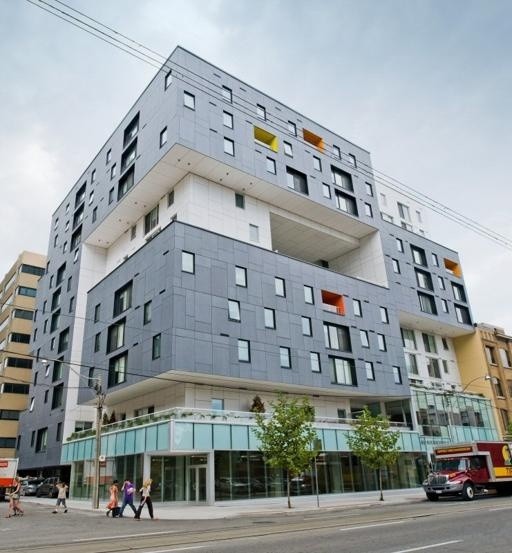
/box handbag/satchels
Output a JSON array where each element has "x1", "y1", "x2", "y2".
[{"x1": 12, "y1": 493, "x2": 19, "y2": 498}]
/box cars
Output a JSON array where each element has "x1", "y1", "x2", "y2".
[
  {"x1": 219, "y1": 476, "x2": 324, "y2": 495},
  {"x1": 21, "y1": 476, "x2": 45, "y2": 496},
  {"x1": 36, "y1": 476, "x2": 66, "y2": 498}
]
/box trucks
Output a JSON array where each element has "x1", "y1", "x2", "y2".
[{"x1": 421, "y1": 440, "x2": 512, "y2": 502}]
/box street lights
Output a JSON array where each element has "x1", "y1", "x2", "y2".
[{"x1": 40, "y1": 359, "x2": 106, "y2": 510}]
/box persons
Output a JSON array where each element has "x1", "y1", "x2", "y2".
[
  {"x1": 134, "y1": 479, "x2": 156, "y2": 519},
  {"x1": 115, "y1": 478, "x2": 137, "y2": 517},
  {"x1": 51, "y1": 481, "x2": 68, "y2": 512},
  {"x1": 8, "y1": 475, "x2": 24, "y2": 516},
  {"x1": 104, "y1": 479, "x2": 119, "y2": 517}
]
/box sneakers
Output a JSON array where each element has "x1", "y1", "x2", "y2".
[
  {"x1": 64, "y1": 509, "x2": 67, "y2": 512},
  {"x1": 52, "y1": 510, "x2": 57, "y2": 512}
]
